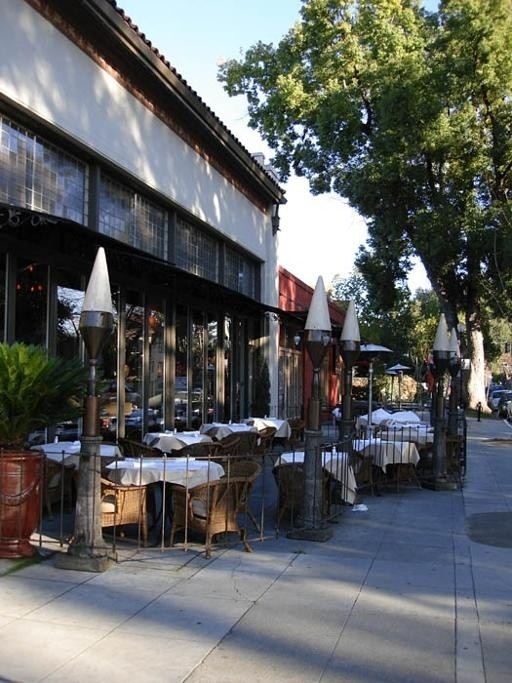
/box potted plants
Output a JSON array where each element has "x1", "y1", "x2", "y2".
[{"x1": 0, "y1": 343, "x2": 105, "y2": 558}]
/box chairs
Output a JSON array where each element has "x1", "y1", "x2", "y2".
[
  {"x1": 219, "y1": 463, "x2": 263, "y2": 538},
  {"x1": 169, "y1": 477, "x2": 255, "y2": 561},
  {"x1": 271, "y1": 462, "x2": 334, "y2": 529},
  {"x1": 73, "y1": 468, "x2": 148, "y2": 553},
  {"x1": 32, "y1": 411, "x2": 433, "y2": 498}
]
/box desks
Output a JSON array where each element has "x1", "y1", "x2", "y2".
[
  {"x1": 273, "y1": 451, "x2": 359, "y2": 507},
  {"x1": 104, "y1": 459, "x2": 227, "y2": 547}
]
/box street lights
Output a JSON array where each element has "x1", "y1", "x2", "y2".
[
  {"x1": 340, "y1": 298, "x2": 360, "y2": 437},
  {"x1": 288, "y1": 275, "x2": 332, "y2": 541},
  {"x1": 52, "y1": 243, "x2": 116, "y2": 574},
  {"x1": 433, "y1": 312, "x2": 460, "y2": 491}
]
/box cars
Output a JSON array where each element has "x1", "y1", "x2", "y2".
[
  {"x1": 487, "y1": 386, "x2": 512, "y2": 421},
  {"x1": 109, "y1": 407, "x2": 158, "y2": 430},
  {"x1": 174, "y1": 390, "x2": 203, "y2": 428},
  {"x1": 28, "y1": 424, "x2": 78, "y2": 446}
]
